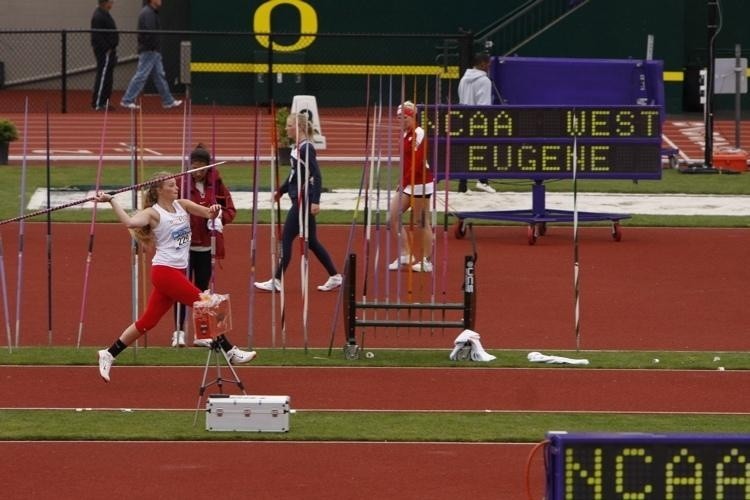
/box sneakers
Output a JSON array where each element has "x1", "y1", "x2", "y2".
[
  {"x1": 96, "y1": 347, "x2": 116, "y2": 384},
  {"x1": 475, "y1": 180, "x2": 498, "y2": 195},
  {"x1": 169, "y1": 331, "x2": 187, "y2": 348},
  {"x1": 317, "y1": 272, "x2": 343, "y2": 293},
  {"x1": 456, "y1": 189, "x2": 478, "y2": 197},
  {"x1": 161, "y1": 99, "x2": 183, "y2": 109},
  {"x1": 226, "y1": 345, "x2": 257, "y2": 365},
  {"x1": 411, "y1": 257, "x2": 433, "y2": 273},
  {"x1": 193, "y1": 337, "x2": 214, "y2": 348},
  {"x1": 89, "y1": 102, "x2": 116, "y2": 112},
  {"x1": 388, "y1": 253, "x2": 415, "y2": 271},
  {"x1": 253, "y1": 278, "x2": 282, "y2": 293},
  {"x1": 120, "y1": 100, "x2": 141, "y2": 109}
]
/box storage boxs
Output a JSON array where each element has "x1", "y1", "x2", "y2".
[{"x1": 205, "y1": 394, "x2": 291, "y2": 433}]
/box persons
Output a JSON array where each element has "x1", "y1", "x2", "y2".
[
  {"x1": 483, "y1": 34, "x2": 498, "y2": 57},
  {"x1": 458, "y1": 52, "x2": 496, "y2": 194},
  {"x1": 254, "y1": 113, "x2": 344, "y2": 294},
  {"x1": 389, "y1": 101, "x2": 434, "y2": 273},
  {"x1": 172, "y1": 142, "x2": 236, "y2": 348},
  {"x1": 119, "y1": 0, "x2": 183, "y2": 113},
  {"x1": 89, "y1": 1, "x2": 119, "y2": 113},
  {"x1": 90, "y1": 171, "x2": 257, "y2": 384}
]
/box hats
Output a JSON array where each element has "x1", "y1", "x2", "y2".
[{"x1": 397, "y1": 100, "x2": 418, "y2": 118}]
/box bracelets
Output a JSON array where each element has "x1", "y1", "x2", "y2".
[{"x1": 108, "y1": 197, "x2": 115, "y2": 203}]
